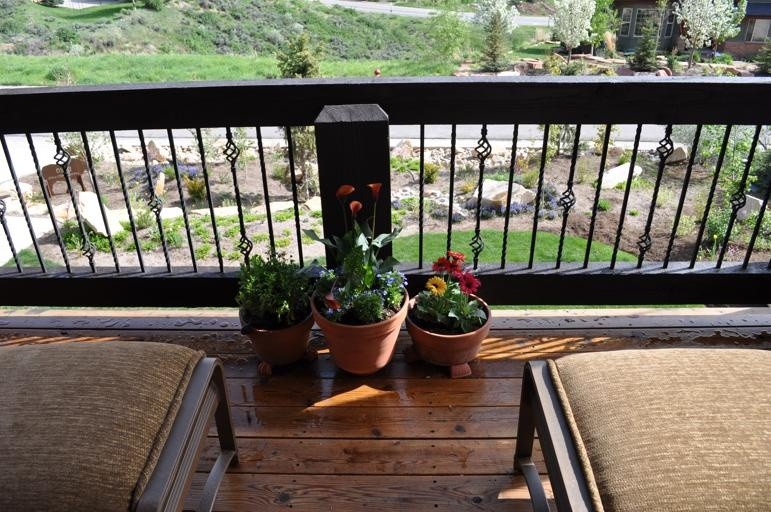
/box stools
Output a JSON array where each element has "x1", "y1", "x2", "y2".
[
  {"x1": 514, "y1": 348, "x2": 771, "y2": 511},
  {"x1": 0, "y1": 342, "x2": 238, "y2": 512}
]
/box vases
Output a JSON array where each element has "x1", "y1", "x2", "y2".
[
  {"x1": 310, "y1": 282, "x2": 407, "y2": 373},
  {"x1": 405, "y1": 290, "x2": 491, "y2": 366}
]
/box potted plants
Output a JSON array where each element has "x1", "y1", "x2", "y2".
[{"x1": 236, "y1": 250, "x2": 312, "y2": 369}]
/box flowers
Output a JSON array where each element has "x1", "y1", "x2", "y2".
[
  {"x1": 413, "y1": 251, "x2": 487, "y2": 330},
  {"x1": 316, "y1": 182, "x2": 408, "y2": 324}
]
